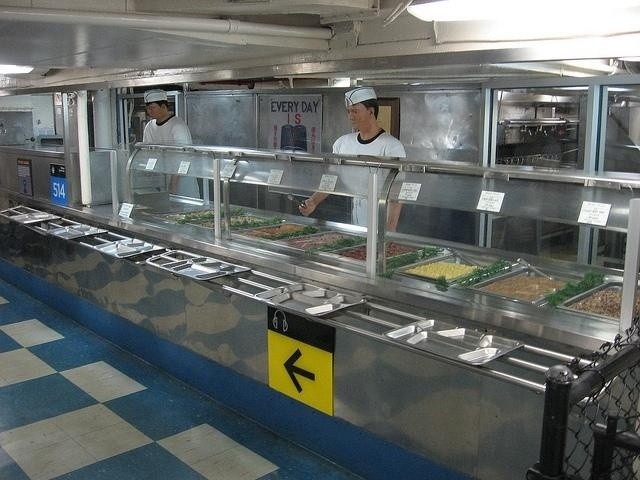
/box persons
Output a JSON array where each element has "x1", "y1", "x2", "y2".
[
  {"x1": 142, "y1": 89, "x2": 200, "y2": 199},
  {"x1": 298, "y1": 86, "x2": 407, "y2": 231}
]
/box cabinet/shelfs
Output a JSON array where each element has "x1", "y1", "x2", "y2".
[{"x1": 123, "y1": 141, "x2": 640, "y2": 349}]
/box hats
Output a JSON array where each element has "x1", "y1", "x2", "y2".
[
  {"x1": 345, "y1": 86, "x2": 378, "y2": 109},
  {"x1": 143, "y1": 89, "x2": 168, "y2": 104}
]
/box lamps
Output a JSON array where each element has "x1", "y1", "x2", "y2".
[{"x1": 0, "y1": 63, "x2": 34, "y2": 74}]
